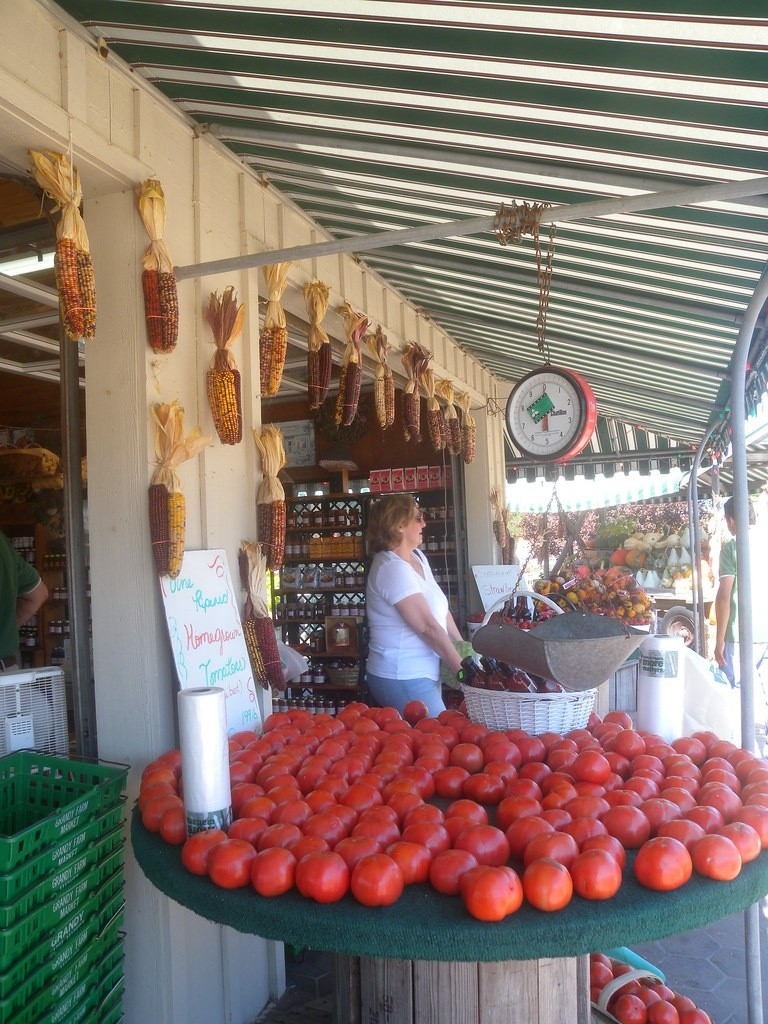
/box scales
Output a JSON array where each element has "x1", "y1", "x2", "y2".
[{"x1": 470, "y1": 362, "x2": 652, "y2": 691}]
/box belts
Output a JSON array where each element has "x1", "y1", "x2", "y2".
[{"x1": 0, "y1": 655, "x2": 17, "y2": 672}]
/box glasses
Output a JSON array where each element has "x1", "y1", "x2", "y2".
[{"x1": 411, "y1": 512, "x2": 423, "y2": 524}]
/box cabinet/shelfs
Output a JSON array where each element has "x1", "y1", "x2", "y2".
[
  {"x1": 271, "y1": 485, "x2": 467, "y2": 692},
  {"x1": 0, "y1": 522, "x2": 92, "y2": 669}
]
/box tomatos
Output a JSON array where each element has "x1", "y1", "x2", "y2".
[
  {"x1": 588, "y1": 952, "x2": 712, "y2": 1024},
  {"x1": 468, "y1": 611, "x2": 651, "y2": 629},
  {"x1": 138, "y1": 699, "x2": 768, "y2": 921}
]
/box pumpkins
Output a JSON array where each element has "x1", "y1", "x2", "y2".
[{"x1": 532, "y1": 525, "x2": 711, "y2": 617}]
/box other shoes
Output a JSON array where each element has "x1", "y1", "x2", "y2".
[{"x1": 754, "y1": 723, "x2": 768, "y2": 758}]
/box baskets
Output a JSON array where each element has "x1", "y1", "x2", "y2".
[
  {"x1": 456, "y1": 678, "x2": 597, "y2": 737},
  {"x1": 0, "y1": 748, "x2": 132, "y2": 1024}
]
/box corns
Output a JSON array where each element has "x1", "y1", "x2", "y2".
[
  {"x1": 148, "y1": 483, "x2": 186, "y2": 579},
  {"x1": 256, "y1": 500, "x2": 286, "y2": 571},
  {"x1": 242, "y1": 616, "x2": 287, "y2": 692},
  {"x1": 53, "y1": 237, "x2": 477, "y2": 465},
  {"x1": 493, "y1": 520, "x2": 515, "y2": 565}
]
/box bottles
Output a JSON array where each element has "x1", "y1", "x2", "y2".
[
  {"x1": 457, "y1": 654, "x2": 567, "y2": 701},
  {"x1": 503, "y1": 596, "x2": 531, "y2": 622}
]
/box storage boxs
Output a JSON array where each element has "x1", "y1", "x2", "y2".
[
  {"x1": 442, "y1": 465, "x2": 453, "y2": 488},
  {"x1": 429, "y1": 466, "x2": 442, "y2": 489},
  {"x1": 381, "y1": 468, "x2": 393, "y2": 493},
  {"x1": 405, "y1": 467, "x2": 417, "y2": 490},
  {"x1": 417, "y1": 465, "x2": 430, "y2": 489},
  {"x1": 0, "y1": 747, "x2": 133, "y2": 1024},
  {"x1": 392, "y1": 468, "x2": 405, "y2": 492},
  {"x1": 369, "y1": 470, "x2": 381, "y2": 492}
]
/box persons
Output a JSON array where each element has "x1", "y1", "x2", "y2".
[
  {"x1": 0, "y1": 530, "x2": 48, "y2": 671},
  {"x1": 715, "y1": 496, "x2": 768, "y2": 689},
  {"x1": 366, "y1": 493, "x2": 466, "y2": 719}
]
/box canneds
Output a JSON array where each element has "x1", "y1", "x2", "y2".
[
  {"x1": 6, "y1": 536, "x2": 69, "y2": 658},
  {"x1": 273, "y1": 504, "x2": 457, "y2": 717}
]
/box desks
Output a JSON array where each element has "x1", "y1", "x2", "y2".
[{"x1": 131, "y1": 798, "x2": 768, "y2": 1024}]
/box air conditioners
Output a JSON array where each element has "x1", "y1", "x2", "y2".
[{"x1": 0, "y1": 666, "x2": 70, "y2": 778}]
